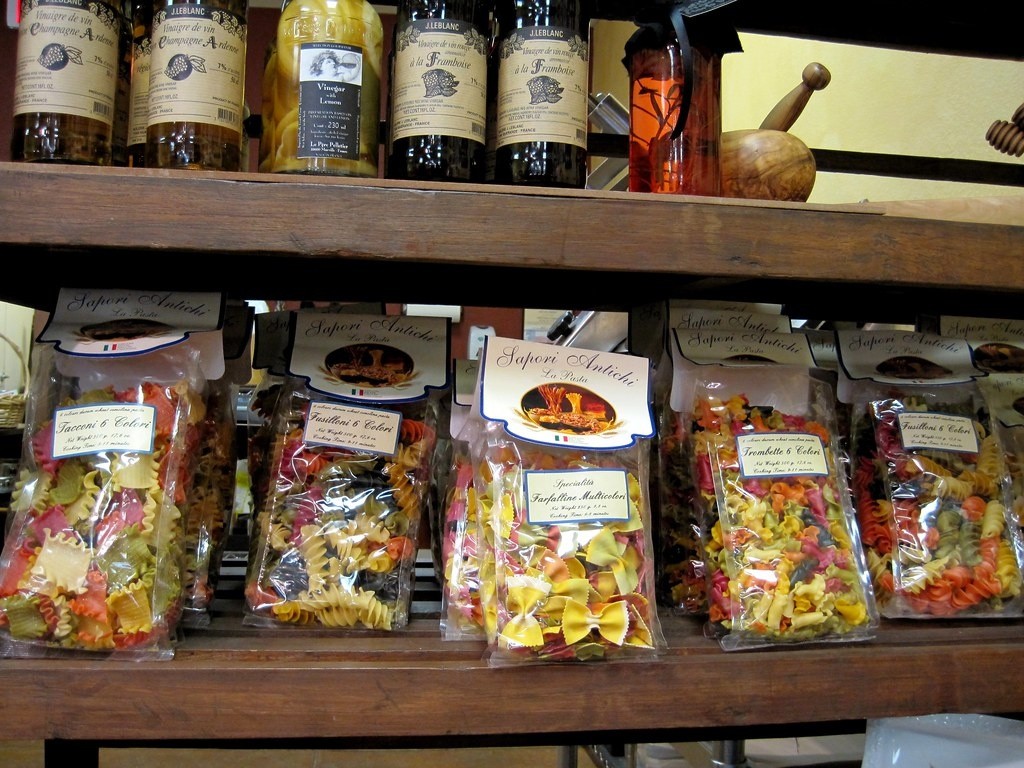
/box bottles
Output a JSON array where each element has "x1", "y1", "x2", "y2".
[
  {"x1": 388, "y1": 0, "x2": 488, "y2": 182},
  {"x1": 256, "y1": 0, "x2": 384, "y2": 175},
  {"x1": 11, "y1": 0, "x2": 246, "y2": 178},
  {"x1": 498, "y1": 0, "x2": 590, "y2": 188},
  {"x1": 629, "y1": 28, "x2": 722, "y2": 197}
]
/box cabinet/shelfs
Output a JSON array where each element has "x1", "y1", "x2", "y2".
[{"x1": 0, "y1": 160, "x2": 1024, "y2": 738}]
